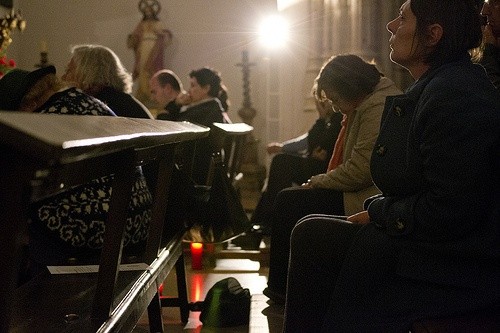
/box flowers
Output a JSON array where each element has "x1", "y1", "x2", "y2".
[{"x1": 0, "y1": 55, "x2": 16, "y2": 78}]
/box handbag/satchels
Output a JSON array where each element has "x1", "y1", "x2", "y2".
[{"x1": 198, "y1": 277, "x2": 252, "y2": 328}]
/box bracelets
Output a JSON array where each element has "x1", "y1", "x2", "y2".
[
  {"x1": 308, "y1": 178, "x2": 314, "y2": 190},
  {"x1": 302, "y1": 182, "x2": 306, "y2": 188}
]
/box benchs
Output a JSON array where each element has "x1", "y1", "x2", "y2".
[{"x1": 0, "y1": 110, "x2": 255, "y2": 333}]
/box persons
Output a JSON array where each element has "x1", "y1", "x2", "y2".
[
  {"x1": 154, "y1": 67, "x2": 231, "y2": 187},
  {"x1": 149, "y1": 69, "x2": 185, "y2": 108},
  {"x1": 0, "y1": 65, "x2": 153, "y2": 309},
  {"x1": 262, "y1": 53, "x2": 402, "y2": 307},
  {"x1": 283, "y1": 0, "x2": 500, "y2": 333},
  {"x1": 127, "y1": 4, "x2": 173, "y2": 108},
  {"x1": 480, "y1": 0, "x2": 500, "y2": 90},
  {"x1": 232, "y1": 84, "x2": 344, "y2": 253},
  {"x1": 58, "y1": 43, "x2": 190, "y2": 238}
]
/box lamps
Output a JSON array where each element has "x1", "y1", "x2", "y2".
[{"x1": 190, "y1": 242, "x2": 204, "y2": 271}]
